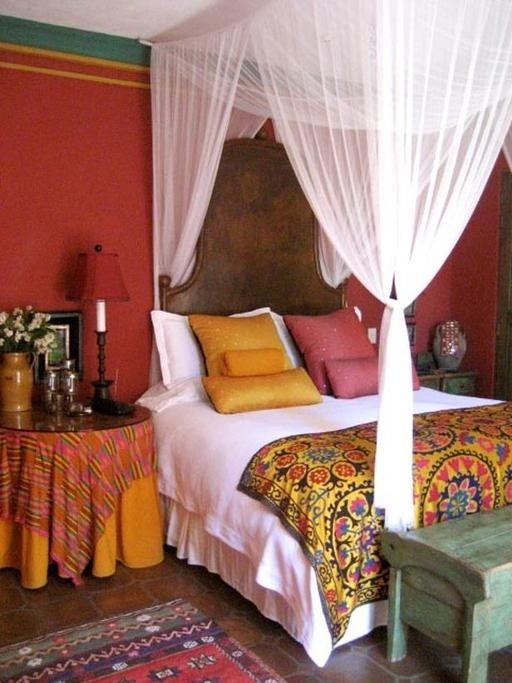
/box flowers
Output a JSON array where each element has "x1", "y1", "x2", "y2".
[{"x1": 0, "y1": 303, "x2": 62, "y2": 356}]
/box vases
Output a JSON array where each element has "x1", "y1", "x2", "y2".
[
  {"x1": 1, "y1": 350, "x2": 37, "y2": 413},
  {"x1": 431, "y1": 319, "x2": 468, "y2": 373}
]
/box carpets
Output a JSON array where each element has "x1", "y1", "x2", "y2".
[{"x1": 0, "y1": 597, "x2": 287, "y2": 683}]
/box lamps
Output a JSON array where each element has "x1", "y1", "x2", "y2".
[{"x1": 66, "y1": 242, "x2": 137, "y2": 415}]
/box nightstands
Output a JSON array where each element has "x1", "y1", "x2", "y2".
[{"x1": 417, "y1": 370, "x2": 480, "y2": 396}]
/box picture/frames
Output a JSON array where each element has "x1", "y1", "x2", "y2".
[
  {"x1": 406, "y1": 324, "x2": 416, "y2": 346},
  {"x1": 32, "y1": 310, "x2": 84, "y2": 386},
  {"x1": 405, "y1": 300, "x2": 416, "y2": 317}
]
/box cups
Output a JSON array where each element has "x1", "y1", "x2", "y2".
[{"x1": 51, "y1": 393, "x2": 71, "y2": 415}]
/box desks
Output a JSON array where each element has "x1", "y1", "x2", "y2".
[{"x1": 0, "y1": 398, "x2": 167, "y2": 589}]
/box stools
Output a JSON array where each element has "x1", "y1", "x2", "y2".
[{"x1": 378, "y1": 504, "x2": 512, "y2": 683}]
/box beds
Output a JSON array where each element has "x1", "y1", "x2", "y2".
[{"x1": 133, "y1": 137, "x2": 511, "y2": 670}]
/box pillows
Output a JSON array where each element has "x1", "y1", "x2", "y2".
[{"x1": 149, "y1": 306, "x2": 421, "y2": 415}]
